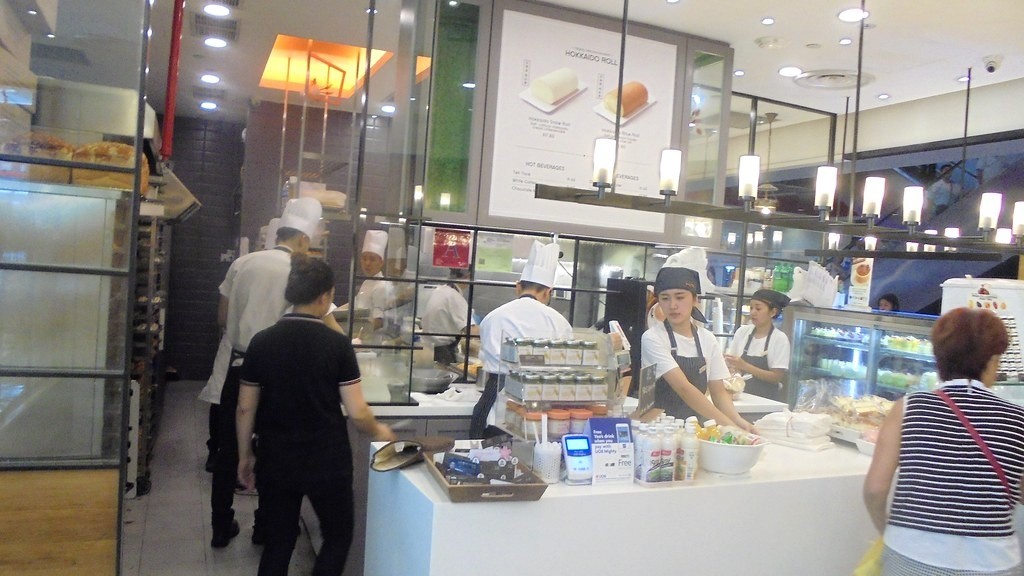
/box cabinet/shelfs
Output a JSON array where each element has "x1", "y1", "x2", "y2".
[
  {"x1": 301, "y1": 419, "x2": 472, "y2": 576},
  {"x1": 496, "y1": 328, "x2": 614, "y2": 439},
  {"x1": 102, "y1": 215, "x2": 165, "y2": 494}
]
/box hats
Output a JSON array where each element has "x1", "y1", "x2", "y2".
[
  {"x1": 519, "y1": 240, "x2": 560, "y2": 289},
  {"x1": 362, "y1": 229, "x2": 388, "y2": 261},
  {"x1": 277, "y1": 197, "x2": 321, "y2": 241},
  {"x1": 387, "y1": 228, "x2": 407, "y2": 260}
]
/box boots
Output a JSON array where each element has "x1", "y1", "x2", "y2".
[
  {"x1": 205, "y1": 439, "x2": 216, "y2": 472},
  {"x1": 253, "y1": 508, "x2": 301, "y2": 544},
  {"x1": 211, "y1": 508, "x2": 239, "y2": 547}
]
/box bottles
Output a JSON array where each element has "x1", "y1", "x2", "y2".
[
  {"x1": 630, "y1": 415, "x2": 763, "y2": 482},
  {"x1": 745, "y1": 262, "x2": 794, "y2": 293}
]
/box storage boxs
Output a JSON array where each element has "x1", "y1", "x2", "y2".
[
  {"x1": 299, "y1": 181, "x2": 346, "y2": 208},
  {"x1": 0, "y1": 0, "x2": 58, "y2": 178}
]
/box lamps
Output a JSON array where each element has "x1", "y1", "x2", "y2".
[
  {"x1": 705, "y1": 156, "x2": 760, "y2": 212},
  {"x1": 878, "y1": 186, "x2": 923, "y2": 235},
  {"x1": 927, "y1": 193, "x2": 1002, "y2": 242},
  {"x1": 964, "y1": 201, "x2": 1024, "y2": 245},
  {"x1": 755, "y1": 113, "x2": 778, "y2": 215},
  {"x1": 769, "y1": 167, "x2": 837, "y2": 221},
  {"x1": 829, "y1": 177, "x2": 885, "y2": 228},
  {"x1": 726, "y1": 227, "x2": 961, "y2": 256},
  {"x1": 650, "y1": 150, "x2": 682, "y2": 206},
  {"x1": 577, "y1": 138, "x2": 616, "y2": 199}
]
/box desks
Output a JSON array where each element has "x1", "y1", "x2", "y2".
[{"x1": 709, "y1": 389, "x2": 790, "y2": 421}]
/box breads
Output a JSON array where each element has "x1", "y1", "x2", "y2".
[
  {"x1": 832, "y1": 394, "x2": 896, "y2": 430},
  {"x1": 534, "y1": 68, "x2": 579, "y2": 105},
  {"x1": 0, "y1": 130, "x2": 149, "y2": 194},
  {"x1": 606, "y1": 82, "x2": 648, "y2": 116}
]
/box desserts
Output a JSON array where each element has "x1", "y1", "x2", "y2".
[{"x1": 809, "y1": 326, "x2": 933, "y2": 387}]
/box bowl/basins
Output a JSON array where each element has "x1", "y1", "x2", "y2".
[{"x1": 698, "y1": 439, "x2": 764, "y2": 474}]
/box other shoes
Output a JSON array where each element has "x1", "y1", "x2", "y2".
[{"x1": 235, "y1": 488, "x2": 259, "y2": 496}]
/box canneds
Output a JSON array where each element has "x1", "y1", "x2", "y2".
[
  {"x1": 504, "y1": 337, "x2": 599, "y2": 366},
  {"x1": 504, "y1": 370, "x2": 607, "y2": 402},
  {"x1": 504, "y1": 400, "x2": 607, "y2": 438}
]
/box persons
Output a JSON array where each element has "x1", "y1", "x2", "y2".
[
  {"x1": 198, "y1": 197, "x2": 349, "y2": 549},
  {"x1": 204, "y1": 217, "x2": 282, "y2": 495},
  {"x1": 863, "y1": 306, "x2": 1023, "y2": 576},
  {"x1": 647, "y1": 247, "x2": 716, "y2": 329},
  {"x1": 470, "y1": 240, "x2": 572, "y2": 440},
  {"x1": 918, "y1": 163, "x2": 937, "y2": 185},
  {"x1": 724, "y1": 289, "x2": 791, "y2": 424},
  {"x1": 641, "y1": 267, "x2": 762, "y2": 437},
  {"x1": 256, "y1": 218, "x2": 281, "y2": 250},
  {"x1": 926, "y1": 164, "x2": 962, "y2": 222},
  {"x1": 238, "y1": 252, "x2": 399, "y2": 576},
  {"x1": 382, "y1": 225, "x2": 417, "y2": 346},
  {"x1": 331, "y1": 230, "x2": 399, "y2": 337},
  {"x1": 878, "y1": 293, "x2": 903, "y2": 401},
  {"x1": 421, "y1": 264, "x2": 480, "y2": 364}
]
